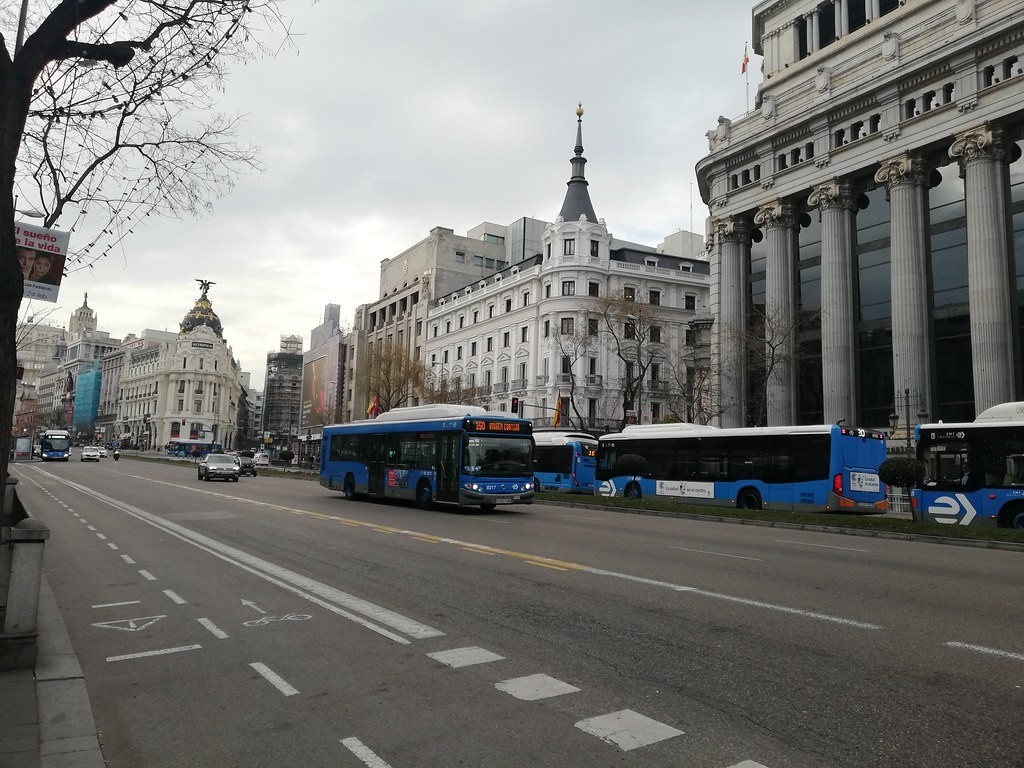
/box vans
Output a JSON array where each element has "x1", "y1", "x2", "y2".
[{"x1": 254, "y1": 453, "x2": 269, "y2": 467}]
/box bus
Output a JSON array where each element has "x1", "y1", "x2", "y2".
[
  {"x1": 40, "y1": 430, "x2": 69, "y2": 462},
  {"x1": 167, "y1": 439, "x2": 222, "y2": 457},
  {"x1": 532, "y1": 432, "x2": 597, "y2": 496},
  {"x1": 593, "y1": 422, "x2": 888, "y2": 515},
  {"x1": 319, "y1": 403, "x2": 535, "y2": 512},
  {"x1": 913, "y1": 401, "x2": 1024, "y2": 532}
]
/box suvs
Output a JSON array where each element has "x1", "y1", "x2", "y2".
[
  {"x1": 80, "y1": 447, "x2": 101, "y2": 462},
  {"x1": 98, "y1": 447, "x2": 107, "y2": 457}
]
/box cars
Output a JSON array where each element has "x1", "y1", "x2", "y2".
[{"x1": 198, "y1": 454, "x2": 239, "y2": 482}]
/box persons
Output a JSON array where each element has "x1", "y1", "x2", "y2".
[
  {"x1": 26, "y1": 428, "x2": 325, "y2": 465},
  {"x1": 16, "y1": 245, "x2": 35, "y2": 281},
  {"x1": 29, "y1": 250, "x2": 58, "y2": 286}
]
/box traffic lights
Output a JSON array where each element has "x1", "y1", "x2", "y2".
[{"x1": 182, "y1": 419, "x2": 185, "y2": 425}]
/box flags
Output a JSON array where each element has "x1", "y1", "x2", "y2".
[{"x1": 741, "y1": 45, "x2": 750, "y2": 75}]
